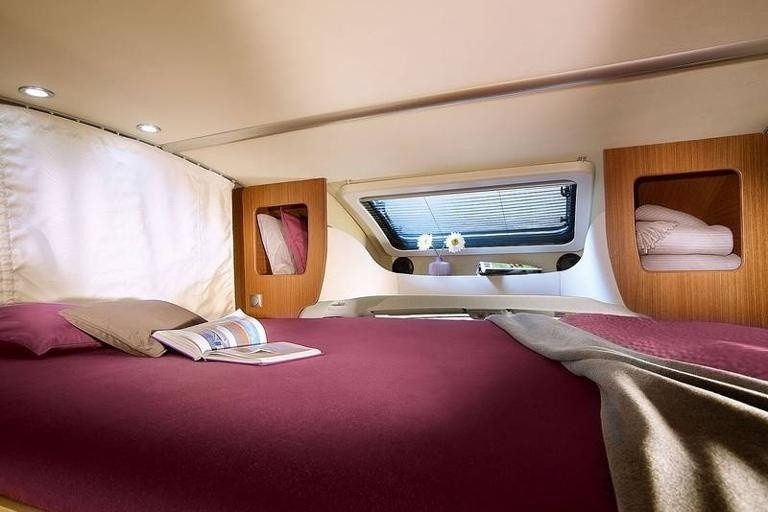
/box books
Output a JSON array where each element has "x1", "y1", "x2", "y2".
[
  {"x1": 476, "y1": 261, "x2": 544, "y2": 275},
  {"x1": 150, "y1": 307, "x2": 323, "y2": 366}
]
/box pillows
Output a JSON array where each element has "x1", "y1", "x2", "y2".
[
  {"x1": 0, "y1": 299, "x2": 209, "y2": 357},
  {"x1": 256, "y1": 209, "x2": 308, "y2": 274}
]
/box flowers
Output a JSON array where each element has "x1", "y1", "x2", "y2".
[{"x1": 416, "y1": 232, "x2": 465, "y2": 257}]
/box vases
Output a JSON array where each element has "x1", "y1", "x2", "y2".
[{"x1": 428, "y1": 257, "x2": 449, "y2": 275}]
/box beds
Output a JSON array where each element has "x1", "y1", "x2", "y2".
[{"x1": 0, "y1": 315, "x2": 767, "y2": 512}]
